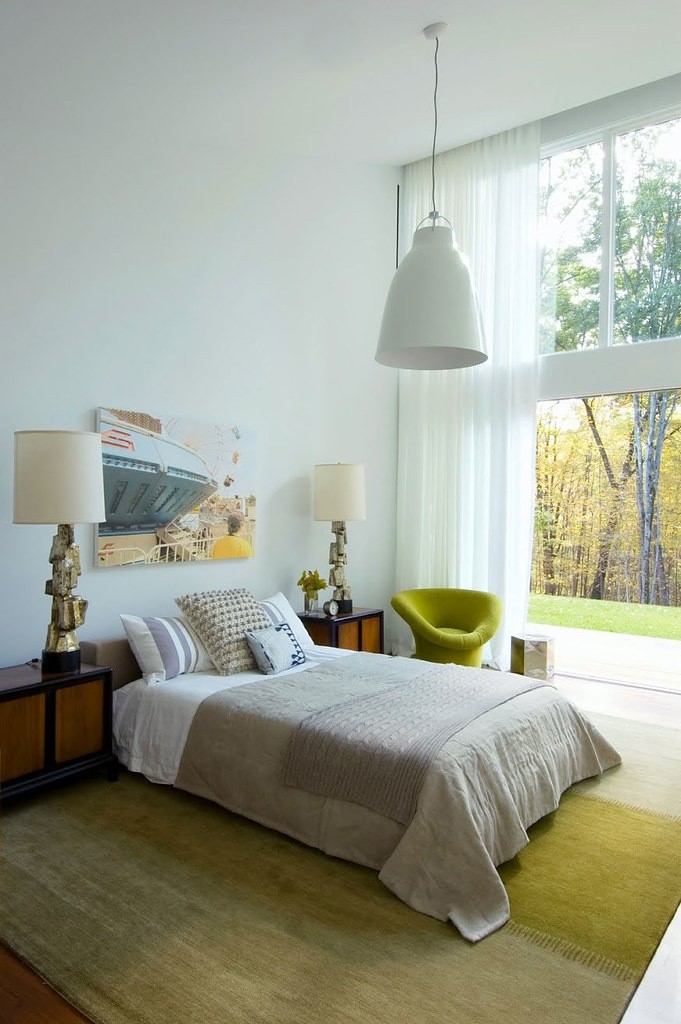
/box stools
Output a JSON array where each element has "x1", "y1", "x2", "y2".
[{"x1": 511, "y1": 634, "x2": 553, "y2": 682}]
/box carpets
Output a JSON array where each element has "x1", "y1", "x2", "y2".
[{"x1": 0, "y1": 706, "x2": 679, "y2": 1024}]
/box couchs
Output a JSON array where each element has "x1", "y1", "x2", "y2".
[{"x1": 391, "y1": 590, "x2": 502, "y2": 669}]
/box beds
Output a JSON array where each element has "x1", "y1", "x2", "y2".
[{"x1": 79, "y1": 633, "x2": 622, "y2": 943}]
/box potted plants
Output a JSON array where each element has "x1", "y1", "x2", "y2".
[{"x1": 296, "y1": 570, "x2": 327, "y2": 614}]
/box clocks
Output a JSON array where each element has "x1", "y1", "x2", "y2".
[{"x1": 323, "y1": 601, "x2": 338, "y2": 615}]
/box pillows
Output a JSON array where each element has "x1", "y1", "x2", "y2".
[
  {"x1": 117, "y1": 614, "x2": 218, "y2": 684},
  {"x1": 245, "y1": 620, "x2": 306, "y2": 676},
  {"x1": 174, "y1": 589, "x2": 272, "y2": 675},
  {"x1": 258, "y1": 592, "x2": 313, "y2": 649}
]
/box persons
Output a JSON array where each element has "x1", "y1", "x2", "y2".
[{"x1": 212, "y1": 510, "x2": 252, "y2": 560}]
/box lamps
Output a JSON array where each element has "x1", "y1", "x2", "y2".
[
  {"x1": 12, "y1": 429, "x2": 107, "y2": 673},
  {"x1": 373, "y1": 21, "x2": 489, "y2": 371},
  {"x1": 312, "y1": 463, "x2": 367, "y2": 613}
]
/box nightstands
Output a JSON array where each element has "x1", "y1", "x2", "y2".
[
  {"x1": 0, "y1": 660, "x2": 121, "y2": 808},
  {"x1": 295, "y1": 604, "x2": 386, "y2": 654}
]
